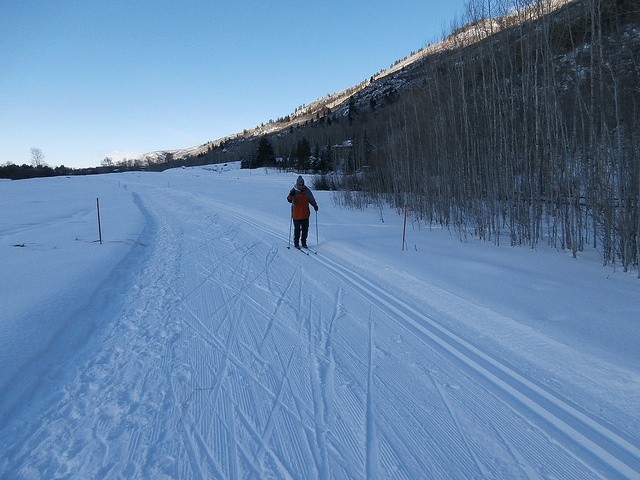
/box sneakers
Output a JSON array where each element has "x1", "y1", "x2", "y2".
[
  {"x1": 294, "y1": 245, "x2": 301, "y2": 250},
  {"x1": 302, "y1": 244, "x2": 308, "y2": 248}
]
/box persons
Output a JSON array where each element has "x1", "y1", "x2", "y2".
[{"x1": 287, "y1": 175, "x2": 318, "y2": 249}]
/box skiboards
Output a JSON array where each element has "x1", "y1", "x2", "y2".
[{"x1": 294, "y1": 245, "x2": 317, "y2": 255}]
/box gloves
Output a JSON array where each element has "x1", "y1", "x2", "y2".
[
  {"x1": 314, "y1": 206, "x2": 318, "y2": 211},
  {"x1": 290, "y1": 190, "x2": 295, "y2": 196}
]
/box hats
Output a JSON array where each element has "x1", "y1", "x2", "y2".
[{"x1": 297, "y1": 176, "x2": 304, "y2": 183}]
